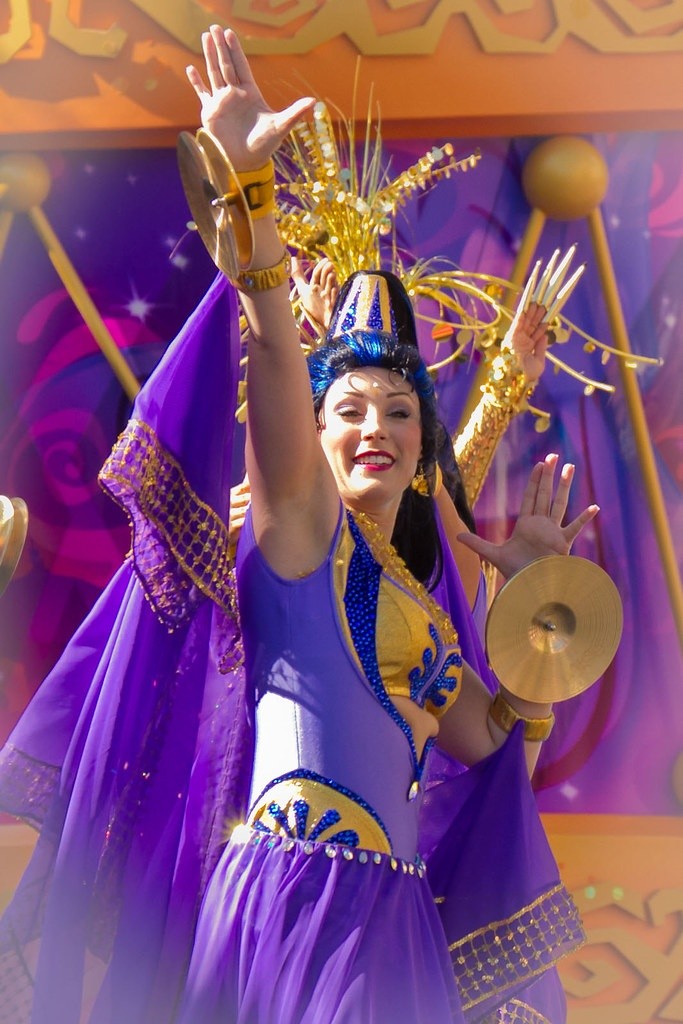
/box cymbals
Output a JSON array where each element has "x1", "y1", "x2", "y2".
[
  {"x1": 485, "y1": 555, "x2": 625, "y2": 704},
  {"x1": 0, "y1": 495, "x2": 30, "y2": 594},
  {"x1": 175, "y1": 129, "x2": 258, "y2": 277}
]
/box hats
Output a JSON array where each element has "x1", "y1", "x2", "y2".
[{"x1": 325, "y1": 266, "x2": 418, "y2": 359}]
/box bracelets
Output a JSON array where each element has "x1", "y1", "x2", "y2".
[
  {"x1": 488, "y1": 693, "x2": 556, "y2": 742},
  {"x1": 226, "y1": 252, "x2": 296, "y2": 293}
]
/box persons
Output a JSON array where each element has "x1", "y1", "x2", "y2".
[
  {"x1": 182, "y1": 22, "x2": 606, "y2": 1024},
  {"x1": 290, "y1": 253, "x2": 557, "y2": 510}
]
